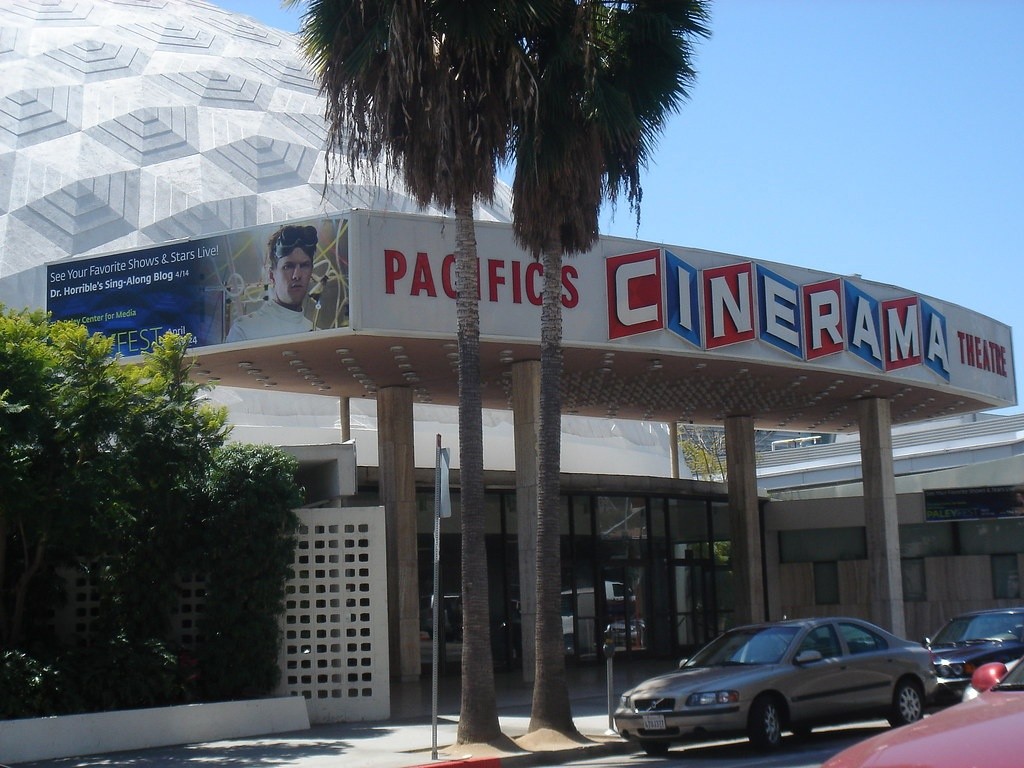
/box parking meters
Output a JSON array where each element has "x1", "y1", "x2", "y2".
[{"x1": 603, "y1": 624, "x2": 616, "y2": 728}]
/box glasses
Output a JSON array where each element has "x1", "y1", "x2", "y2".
[{"x1": 275, "y1": 226, "x2": 318, "y2": 257}]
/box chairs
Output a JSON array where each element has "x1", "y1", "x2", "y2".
[{"x1": 816, "y1": 636, "x2": 839, "y2": 657}]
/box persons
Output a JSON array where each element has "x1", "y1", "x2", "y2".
[{"x1": 223, "y1": 225, "x2": 324, "y2": 343}]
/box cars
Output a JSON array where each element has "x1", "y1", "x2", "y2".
[
  {"x1": 921, "y1": 607, "x2": 1024, "y2": 693},
  {"x1": 823, "y1": 659, "x2": 1024, "y2": 768},
  {"x1": 613, "y1": 616, "x2": 938, "y2": 755}
]
